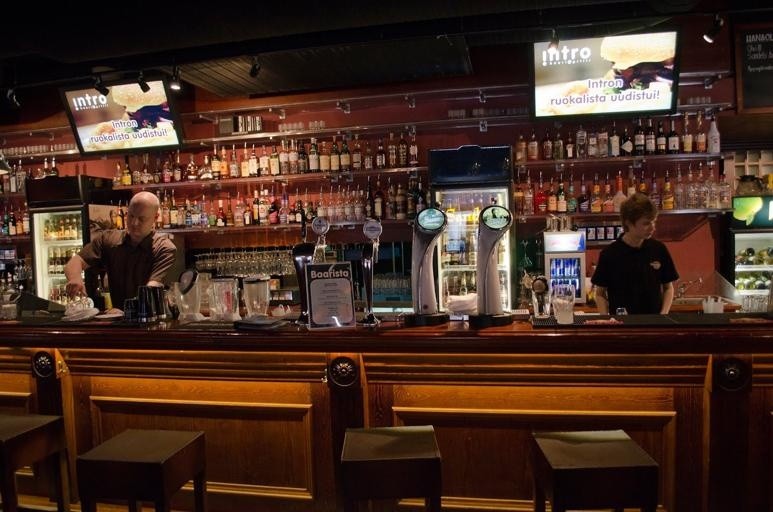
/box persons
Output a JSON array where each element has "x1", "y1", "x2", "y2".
[
  {"x1": 62, "y1": 191, "x2": 178, "y2": 315},
  {"x1": 591, "y1": 193, "x2": 680, "y2": 316}
]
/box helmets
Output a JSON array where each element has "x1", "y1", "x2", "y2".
[{"x1": 93, "y1": 314, "x2": 122, "y2": 322}]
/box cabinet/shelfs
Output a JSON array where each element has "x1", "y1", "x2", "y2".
[
  {"x1": 123, "y1": 285, "x2": 166, "y2": 326},
  {"x1": 42, "y1": 157, "x2": 49, "y2": 178},
  {"x1": 515, "y1": 110, "x2": 731, "y2": 213},
  {"x1": 0, "y1": 159, "x2": 41, "y2": 293},
  {"x1": 49, "y1": 156, "x2": 58, "y2": 176},
  {"x1": 44, "y1": 214, "x2": 84, "y2": 305},
  {"x1": 436, "y1": 195, "x2": 507, "y2": 304}
]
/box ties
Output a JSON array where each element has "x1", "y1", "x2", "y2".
[
  {"x1": 59, "y1": 72, "x2": 183, "y2": 155},
  {"x1": 528, "y1": 27, "x2": 681, "y2": 119}
]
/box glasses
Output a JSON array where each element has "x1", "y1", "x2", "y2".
[{"x1": 0, "y1": 7, "x2": 726, "y2": 110}]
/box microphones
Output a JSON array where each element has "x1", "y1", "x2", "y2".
[
  {"x1": 335, "y1": 423, "x2": 444, "y2": 511},
  {"x1": 0, "y1": 408, "x2": 73, "y2": 511},
  {"x1": 71, "y1": 426, "x2": 211, "y2": 511},
  {"x1": 526, "y1": 425, "x2": 664, "y2": 512}
]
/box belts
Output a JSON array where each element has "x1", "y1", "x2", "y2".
[{"x1": 192, "y1": 248, "x2": 298, "y2": 280}]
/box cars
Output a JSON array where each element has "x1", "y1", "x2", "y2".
[
  {"x1": 172, "y1": 277, "x2": 271, "y2": 323},
  {"x1": 742, "y1": 293, "x2": 768, "y2": 312},
  {"x1": 545, "y1": 217, "x2": 574, "y2": 232},
  {"x1": 531, "y1": 275, "x2": 576, "y2": 325}
]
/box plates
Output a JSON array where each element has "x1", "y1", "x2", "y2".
[
  {"x1": 0, "y1": 191, "x2": 30, "y2": 247},
  {"x1": 105, "y1": 164, "x2": 430, "y2": 236},
  {"x1": 508, "y1": 150, "x2": 739, "y2": 221}
]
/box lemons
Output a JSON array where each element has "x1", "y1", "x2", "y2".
[{"x1": 736, "y1": 173, "x2": 765, "y2": 196}]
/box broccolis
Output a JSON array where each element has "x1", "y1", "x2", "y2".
[
  {"x1": 720, "y1": 194, "x2": 772, "y2": 314},
  {"x1": 23, "y1": 174, "x2": 112, "y2": 299},
  {"x1": 423, "y1": 147, "x2": 514, "y2": 312}
]
